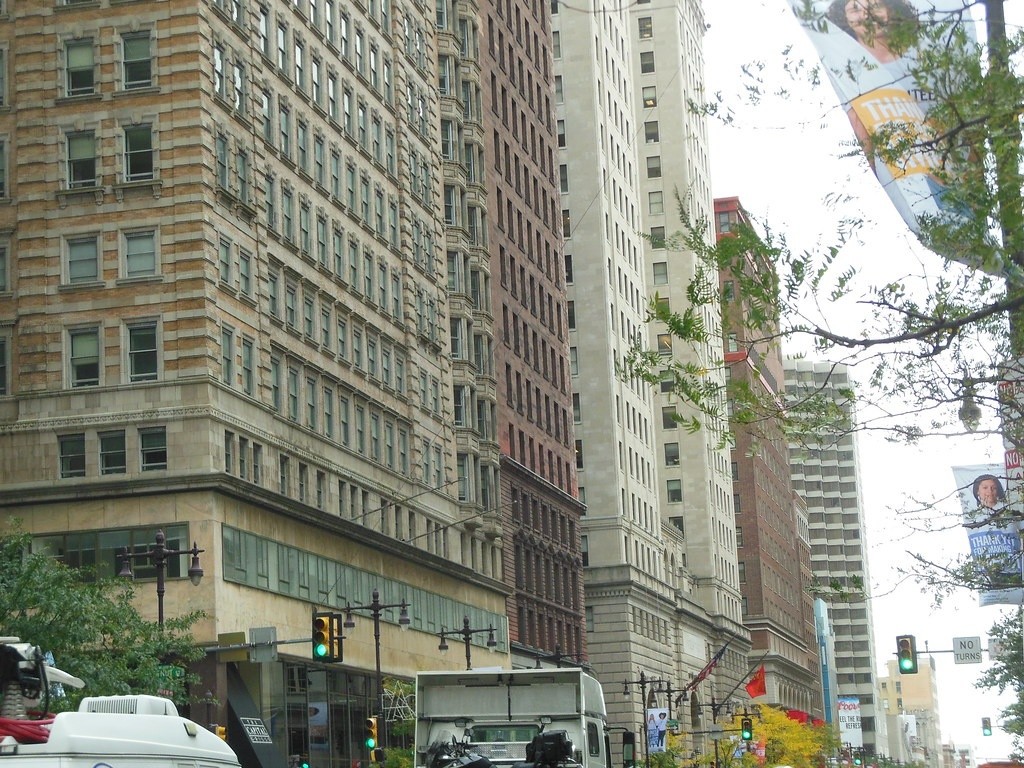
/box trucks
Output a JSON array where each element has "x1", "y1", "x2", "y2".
[{"x1": 415, "y1": 668, "x2": 636, "y2": 768}]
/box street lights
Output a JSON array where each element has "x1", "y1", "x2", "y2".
[
  {"x1": 435, "y1": 615, "x2": 498, "y2": 671},
  {"x1": 679, "y1": 684, "x2": 732, "y2": 768},
  {"x1": 621, "y1": 670, "x2": 689, "y2": 768},
  {"x1": 341, "y1": 588, "x2": 412, "y2": 767},
  {"x1": 116, "y1": 529, "x2": 205, "y2": 659}
]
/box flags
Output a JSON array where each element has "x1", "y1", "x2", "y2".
[
  {"x1": 745, "y1": 663, "x2": 767, "y2": 699},
  {"x1": 691, "y1": 646, "x2": 725, "y2": 689},
  {"x1": 786, "y1": 710, "x2": 824, "y2": 727}
]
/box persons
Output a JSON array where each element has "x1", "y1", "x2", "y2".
[
  {"x1": 973, "y1": 474, "x2": 1006, "y2": 508},
  {"x1": 657, "y1": 712, "x2": 667, "y2": 747},
  {"x1": 647, "y1": 714, "x2": 656, "y2": 747}
]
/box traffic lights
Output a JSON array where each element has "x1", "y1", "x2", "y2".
[
  {"x1": 216, "y1": 727, "x2": 226, "y2": 739},
  {"x1": 311, "y1": 612, "x2": 335, "y2": 663},
  {"x1": 741, "y1": 718, "x2": 753, "y2": 741},
  {"x1": 854, "y1": 751, "x2": 862, "y2": 766},
  {"x1": 896, "y1": 634, "x2": 917, "y2": 674},
  {"x1": 370, "y1": 749, "x2": 382, "y2": 763},
  {"x1": 365, "y1": 718, "x2": 378, "y2": 750},
  {"x1": 981, "y1": 717, "x2": 992, "y2": 736},
  {"x1": 301, "y1": 762, "x2": 309, "y2": 768}
]
